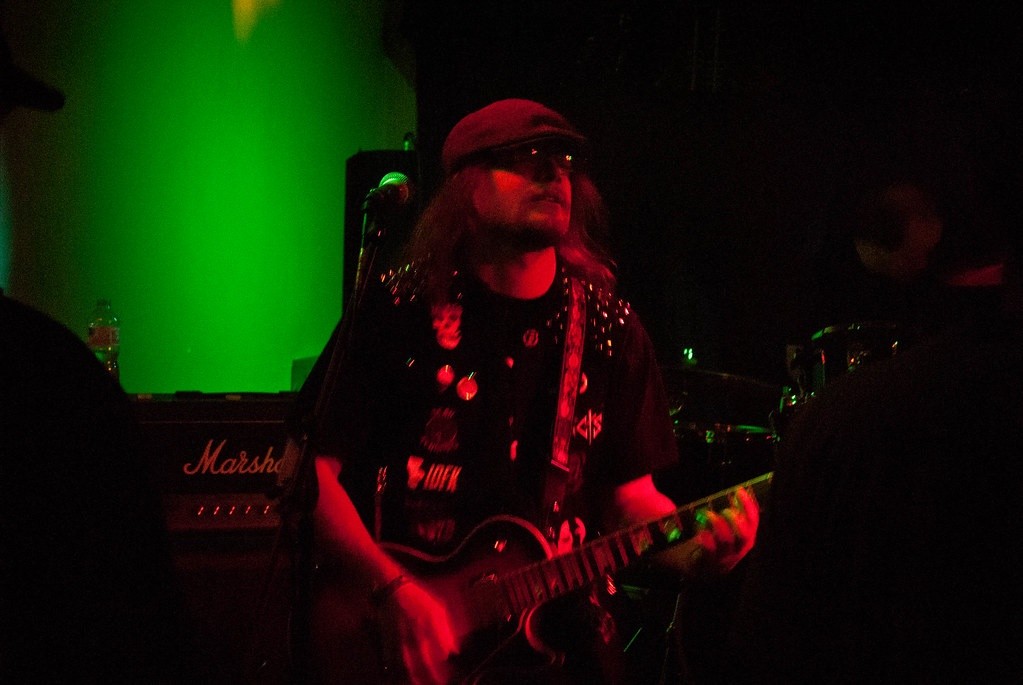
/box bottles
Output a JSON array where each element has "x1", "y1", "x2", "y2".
[{"x1": 88, "y1": 300, "x2": 118, "y2": 382}]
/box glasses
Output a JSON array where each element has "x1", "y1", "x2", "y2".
[{"x1": 482, "y1": 145, "x2": 577, "y2": 173}]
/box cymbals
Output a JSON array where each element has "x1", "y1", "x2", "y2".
[
  {"x1": 671, "y1": 415, "x2": 774, "y2": 438},
  {"x1": 941, "y1": 260, "x2": 1003, "y2": 289},
  {"x1": 678, "y1": 362, "x2": 779, "y2": 398}
]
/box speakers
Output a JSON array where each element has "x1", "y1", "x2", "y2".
[{"x1": 171, "y1": 536, "x2": 410, "y2": 685}]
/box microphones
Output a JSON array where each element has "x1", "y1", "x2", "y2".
[{"x1": 365, "y1": 171, "x2": 415, "y2": 214}]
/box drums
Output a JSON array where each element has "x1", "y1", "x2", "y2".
[{"x1": 811, "y1": 319, "x2": 908, "y2": 395}]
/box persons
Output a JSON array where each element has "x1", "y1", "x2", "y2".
[
  {"x1": 312, "y1": 98, "x2": 761, "y2": 685},
  {"x1": 729, "y1": 184, "x2": 945, "y2": 393},
  {"x1": 0, "y1": 296, "x2": 198, "y2": 685}
]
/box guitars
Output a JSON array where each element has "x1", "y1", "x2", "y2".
[{"x1": 285, "y1": 470, "x2": 781, "y2": 685}]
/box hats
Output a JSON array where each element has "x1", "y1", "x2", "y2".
[{"x1": 441, "y1": 97, "x2": 587, "y2": 169}]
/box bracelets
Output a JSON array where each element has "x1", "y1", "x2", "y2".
[{"x1": 386, "y1": 574, "x2": 412, "y2": 593}]
